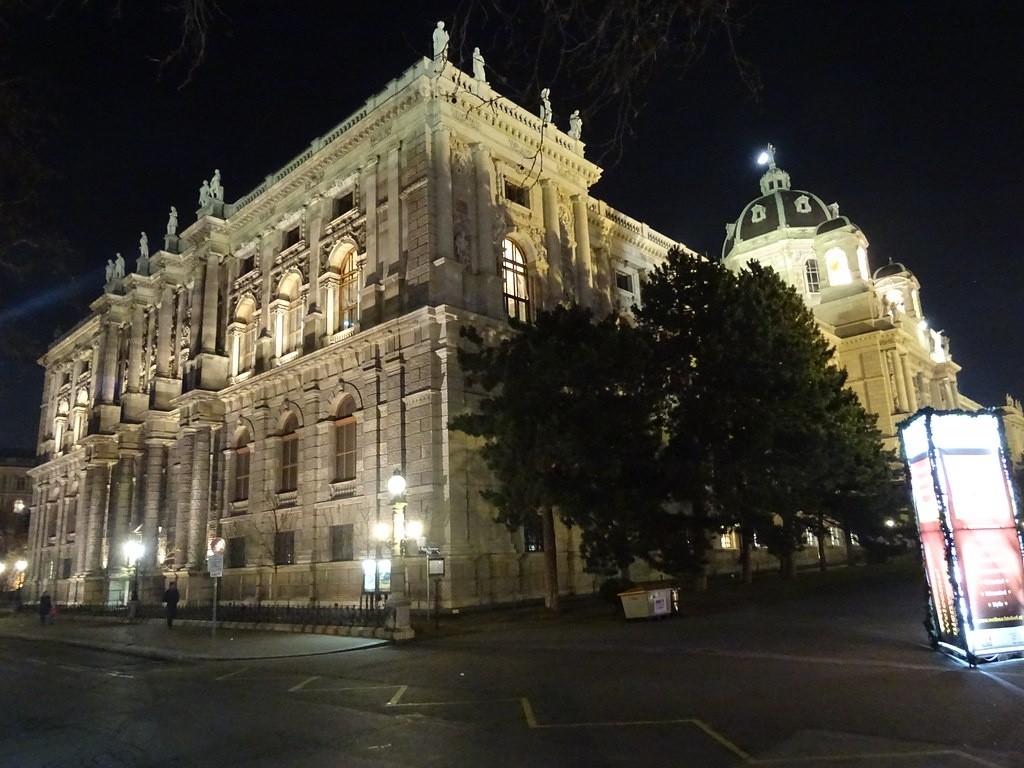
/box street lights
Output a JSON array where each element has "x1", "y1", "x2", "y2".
[
  {"x1": 17, "y1": 561, "x2": 26, "y2": 602},
  {"x1": 127, "y1": 545, "x2": 143, "y2": 619},
  {"x1": 386, "y1": 468, "x2": 415, "y2": 640}
]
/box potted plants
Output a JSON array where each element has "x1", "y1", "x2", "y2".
[
  {"x1": 719, "y1": 570, "x2": 742, "y2": 588},
  {"x1": 599, "y1": 577, "x2": 636, "y2": 614}
]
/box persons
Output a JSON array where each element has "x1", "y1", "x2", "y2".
[
  {"x1": 433, "y1": 21, "x2": 449, "y2": 61},
  {"x1": 569, "y1": 110, "x2": 583, "y2": 139},
  {"x1": 943, "y1": 336, "x2": 950, "y2": 355},
  {"x1": 541, "y1": 89, "x2": 552, "y2": 122},
  {"x1": 209, "y1": 169, "x2": 221, "y2": 199},
  {"x1": 767, "y1": 143, "x2": 776, "y2": 164},
  {"x1": 473, "y1": 47, "x2": 485, "y2": 82},
  {"x1": 891, "y1": 302, "x2": 899, "y2": 315},
  {"x1": 139, "y1": 232, "x2": 149, "y2": 258},
  {"x1": 163, "y1": 582, "x2": 180, "y2": 630},
  {"x1": 38, "y1": 591, "x2": 57, "y2": 626},
  {"x1": 105, "y1": 253, "x2": 126, "y2": 283},
  {"x1": 167, "y1": 206, "x2": 179, "y2": 235},
  {"x1": 199, "y1": 180, "x2": 210, "y2": 206}
]
